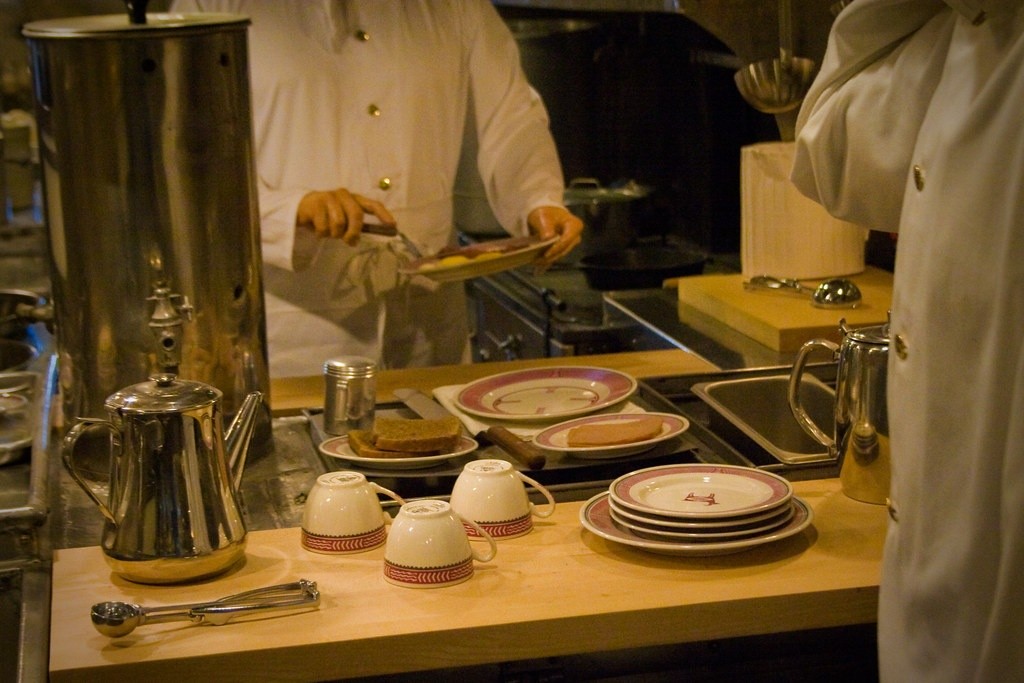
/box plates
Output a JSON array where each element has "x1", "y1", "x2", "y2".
[
  {"x1": 532, "y1": 412, "x2": 690, "y2": 458},
  {"x1": 397, "y1": 235, "x2": 561, "y2": 282},
  {"x1": 609, "y1": 463, "x2": 793, "y2": 518},
  {"x1": 609, "y1": 508, "x2": 794, "y2": 537},
  {"x1": 609, "y1": 497, "x2": 792, "y2": 527},
  {"x1": 579, "y1": 490, "x2": 815, "y2": 556},
  {"x1": 318, "y1": 434, "x2": 479, "y2": 470},
  {"x1": 452, "y1": 365, "x2": 637, "y2": 422}
]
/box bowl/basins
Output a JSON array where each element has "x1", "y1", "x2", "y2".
[{"x1": 578, "y1": 246, "x2": 706, "y2": 292}]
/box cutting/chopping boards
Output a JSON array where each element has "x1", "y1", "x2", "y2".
[{"x1": 678, "y1": 266, "x2": 894, "y2": 352}]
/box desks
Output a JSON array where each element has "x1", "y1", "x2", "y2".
[{"x1": 49, "y1": 348, "x2": 878, "y2": 683}]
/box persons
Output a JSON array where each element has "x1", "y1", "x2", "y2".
[
  {"x1": 166, "y1": 0, "x2": 583, "y2": 380},
  {"x1": 790, "y1": 2, "x2": 1024, "y2": 683}
]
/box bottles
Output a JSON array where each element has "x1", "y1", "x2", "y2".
[{"x1": 323, "y1": 356, "x2": 377, "y2": 435}]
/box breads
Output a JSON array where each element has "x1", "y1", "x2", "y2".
[
  {"x1": 567, "y1": 419, "x2": 664, "y2": 447},
  {"x1": 346, "y1": 418, "x2": 463, "y2": 458}
]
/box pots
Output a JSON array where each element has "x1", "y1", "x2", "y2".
[{"x1": 563, "y1": 178, "x2": 646, "y2": 267}]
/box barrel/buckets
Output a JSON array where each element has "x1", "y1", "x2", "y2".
[{"x1": 742, "y1": 142, "x2": 870, "y2": 279}]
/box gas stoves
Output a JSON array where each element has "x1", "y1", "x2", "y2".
[{"x1": 450, "y1": 202, "x2": 740, "y2": 346}]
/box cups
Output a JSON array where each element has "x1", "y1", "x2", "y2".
[
  {"x1": 301, "y1": 471, "x2": 406, "y2": 555},
  {"x1": 384, "y1": 499, "x2": 497, "y2": 590},
  {"x1": 449, "y1": 458, "x2": 555, "y2": 541}
]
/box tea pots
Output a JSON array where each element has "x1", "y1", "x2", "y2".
[
  {"x1": 62, "y1": 373, "x2": 265, "y2": 585},
  {"x1": 787, "y1": 310, "x2": 892, "y2": 505}
]
